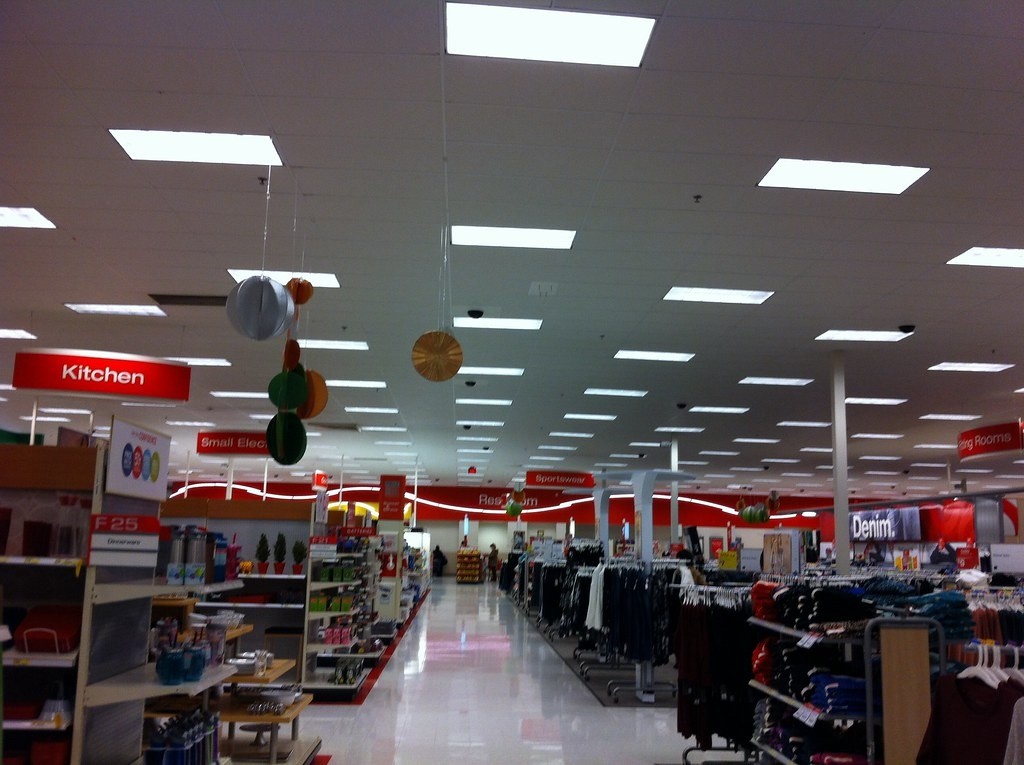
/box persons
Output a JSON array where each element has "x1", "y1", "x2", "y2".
[
  {"x1": 515, "y1": 533, "x2": 523, "y2": 548},
  {"x1": 488, "y1": 543, "x2": 498, "y2": 581},
  {"x1": 851, "y1": 553, "x2": 866, "y2": 567},
  {"x1": 433, "y1": 545, "x2": 444, "y2": 577}
]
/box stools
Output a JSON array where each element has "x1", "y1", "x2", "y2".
[{"x1": 265, "y1": 626, "x2": 303, "y2": 684}]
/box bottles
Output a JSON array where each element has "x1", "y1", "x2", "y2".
[{"x1": 144, "y1": 705, "x2": 220, "y2": 765}]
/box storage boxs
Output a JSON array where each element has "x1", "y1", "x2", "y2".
[
  {"x1": 320, "y1": 566, "x2": 357, "y2": 581},
  {"x1": 326, "y1": 628, "x2": 351, "y2": 645},
  {"x1": 332, "y1": 657, "x2": 365, "y2": 684},
  {"x1": 309, "y1": 593, "x2": 359, "y2": 612}
]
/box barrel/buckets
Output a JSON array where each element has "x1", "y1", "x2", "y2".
[{"x1": 187, "y1": 532, "x2": 216, "y2": 583}]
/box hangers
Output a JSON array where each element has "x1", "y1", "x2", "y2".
[
  {"x1": 955, "y1": 639, "x2": 1024, "y2": 689},
  {"x1": 682, "y1": 585, "x2": 736, "y2": 608},
  {"x1": 761, "y1": 569, "x2": 932, "y2": 588},
  {"x1": 967, "y1": 585, "x2": 1024, "y2": 614},
  {"x1": 599, "y1": 557, "x2": 689, "y2": 571},
  {"x1": 578, "y1": 567, "x2": 596, "y2": 576}
]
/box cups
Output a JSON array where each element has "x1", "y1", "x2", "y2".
[
  {"x1": 157, "y1": 620, "x2": 178, "y2": 648},
  {"x1": 184, "y1": 647, "x2": 203, "y2": 681},
  {"x1": 257, "y1": 653, "x2": 267, "y2": 675},
  {"x1": 227, "y1": 545, "x2": 238, "y2": 580},
  {"x1": 207, "y1": 624, "x2": 226, "y2": 666},
  {"x1": 156, "y1": 648, "x2": 184, "y2": 685}
]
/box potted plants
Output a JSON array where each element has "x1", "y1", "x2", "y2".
[
  {"x1": 273, "y1": 532, "x2": 286, "y2": 574},
  {"x1": 291, "y1": 540, "x2": 308, "y2": 573},
  {"x1": 256, "y1": 534, "x2": 272, "y2": 574}
]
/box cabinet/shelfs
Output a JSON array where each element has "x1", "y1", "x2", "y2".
[
  {"x1": 161, "y1": 491, "x2": 380, "y2": 703},
  {"x1": 749, "y1": 606, "x2": 944, "y2": 765},
  {"x1": 0, "y1": 441, "x2": 162, "y2": 765},
  {"x1": 370, "y1": 531, "x2": 434, "y2": 644},
  {"x1": 147, "y1": 581, "x2": 323, "y2": 765},
  {"x1": 457, "y1": 554, "x2": 480, "y2": 583}
]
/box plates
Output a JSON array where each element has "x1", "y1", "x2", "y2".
[
  {"x1": 225, "y1": 659, "x2": 259, "y2": 675},
  {"x1": 236, "y1": 651, "x2": 274, "y2": 669}
]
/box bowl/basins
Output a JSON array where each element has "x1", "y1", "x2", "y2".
[{"x1": 261, "y1": 690, "x2": 295, "y2": 706}]
